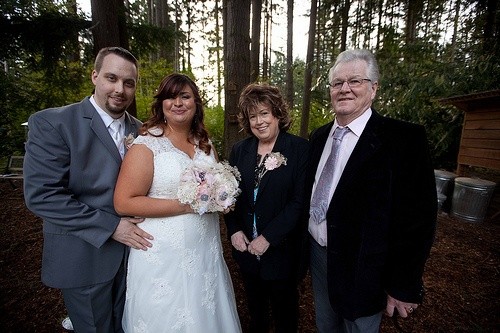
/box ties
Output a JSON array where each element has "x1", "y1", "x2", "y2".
[
  {"x1": 108, "y1": 121, "x2": 125, "y2": 162},
  {"x1": 310, "y1": 128, "x2": 352, "y2": 225}
]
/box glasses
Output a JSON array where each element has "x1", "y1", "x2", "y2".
[{"x1": 328, "y1": 78, "x2": 372, "y2": 88}]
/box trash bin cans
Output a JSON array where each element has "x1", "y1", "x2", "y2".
[
  {"x1": 433, "y1": 170, "x2": 457, "y2": 208},
  {"x1": 451, "y1": 177, "x2": 496, "y2": 223}
]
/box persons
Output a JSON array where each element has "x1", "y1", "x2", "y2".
[
  {"x1": 227, "y1": 82, "x2": 315, "y2": 333},
  {"x1": 308, "y1": 49, "x2": 439, "y2": 333},
  {"x1": 113, "y1": 73, "x2": 242, "y2": 333},
  {"x1": 22, "y1": 47, "x2": 154, "y2": 332}
]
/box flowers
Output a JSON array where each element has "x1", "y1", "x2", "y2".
[
  {"x1": 177, "y1": 161, "x2": 242, "y2": 215},
  {"x1": 264, "y1": 152, "x2": 288, "y2": 171}
]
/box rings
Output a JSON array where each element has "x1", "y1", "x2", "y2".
[{"x1": 408, "y1": 307, "x2": 413, "y2": 314}]
